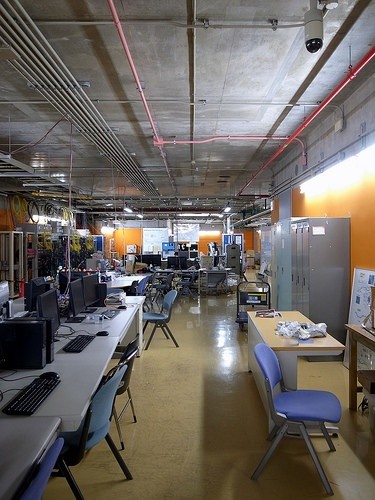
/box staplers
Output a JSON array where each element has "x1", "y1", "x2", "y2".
[{"x1": 255, "y1": 309, "x2": 275, "y2": 318}]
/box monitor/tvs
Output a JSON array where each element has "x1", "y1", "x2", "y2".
[
  {"x1": 168, "y1": 257, "x2": 187, "y2": 270},
  {"x1": 0, "y1": 281, "x2": 14, "y2": 321},
  {"x1": 141, "y1": 255, "x2": 161, "y2": 271},
  {"x1": 25, "y1": 271, "x2": 100, "y2": 343}
]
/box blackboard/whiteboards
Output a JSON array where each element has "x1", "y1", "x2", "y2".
[{"x1": 343, "y1": 267, "x2": 375, "y2": 372}]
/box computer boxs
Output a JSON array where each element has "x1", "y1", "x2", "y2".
[{"x1": 0, "y1": 316, "x2": 54, "y2": 370}]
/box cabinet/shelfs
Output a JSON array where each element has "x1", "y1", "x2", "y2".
[
  {"x1": 237, "y1": 280, "x2": 270, "y2": 331},
  {"x1": 258, "y1": 217, "x2": 349, "y2": 363}
]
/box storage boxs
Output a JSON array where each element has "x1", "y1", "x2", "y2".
[{"x1": 355, "y1": 370, "x2": 375, "y2": 440}]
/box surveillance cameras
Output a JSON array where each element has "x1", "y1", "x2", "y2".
[{"x1": 304, "y1": 9, "x2": 324, "y2": 53}]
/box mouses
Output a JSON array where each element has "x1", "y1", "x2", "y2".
[
  {"x1": 39, "y1": 372, "x2": 60, "y2": 379},
  {"x1": 96, "y1": 331, "x2": 109, "y2": 336},
  {"x1": 118, "y1": 305, "x2": 127, "y2": 309}
]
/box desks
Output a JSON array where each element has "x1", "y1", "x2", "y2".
[
  {"x1": 247, "y1": 310, "x2": 346, "y2": 418},
  {"x1": 344, "y1": 323, "x2": 375, "y2": 411},
  {"x1": 99, "y1": 264, "x2": 237, "y2": 291},
  {"x1": 0, "y1": 337, "x2": 120, "y2": 431},
  {"x1": 0, "y1": 415, "x2": 62, "y2": 500},
  {"x1": 56, "y1": 295, "x2": 146, "y2": 359}
]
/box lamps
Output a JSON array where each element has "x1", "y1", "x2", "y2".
[
  {"x1": 124, "y1": 202, "x2": 144, "y2": 220},
  {"x1": 224, "y1": 203, "x2": 231, "y2": 214}
]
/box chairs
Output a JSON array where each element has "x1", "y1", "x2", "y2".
[
  {"x1": 19, "y1": 270, "x2": 233, "y2": 500},
  {"x1": 240, "y1": 261, "x2": 250, "y2": 283},
  {"x1": 252, "y1": 341, "x2": 342, "y2": 496}
]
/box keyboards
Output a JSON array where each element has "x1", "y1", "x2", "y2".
[
  {"x1": 100, "y1": 310, "x2": 119, "y2": 319},
  {"x1": 63, "y1": 335, "x2": 95, "y2": 353},
  {"x1": 2, "y1": 379, "x2": 61, "y2": 415}
]
